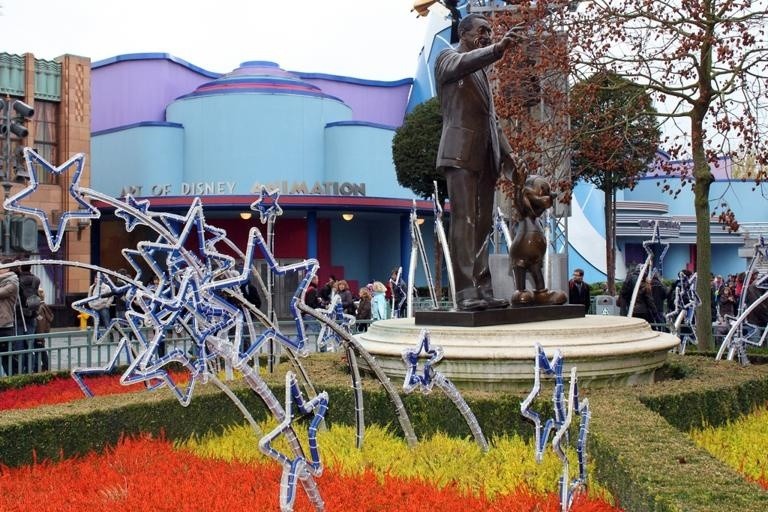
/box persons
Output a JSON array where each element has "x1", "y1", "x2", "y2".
[
  {"x1": 0, "y1": 255, "x2": 54, "y2": 375},
  {"x1": 617, "y1": 263, "x2": 768, "y2": 330},
  {"x1": 88, "y1": 274, "x2": 115, "y2": 342},
  {"x1": 435, "y1": 13, "x2": 527, "y2": 311},
  {"x1": 116, "y1": 268, "x2": 133, "y2": 341},
  {"x1": 153, "y1": 278, "x2": 159, "y2": 286},
  {"x1": 568, "y1": 269, "x2": 591, "y2": 314},
  {"x1": 304, "y1": 267, "x2": 408, "y2": 332}
]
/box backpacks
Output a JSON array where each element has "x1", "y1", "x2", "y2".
[{"x1": 19, "y1": 275, "x2": 40, "y2": 319}]
